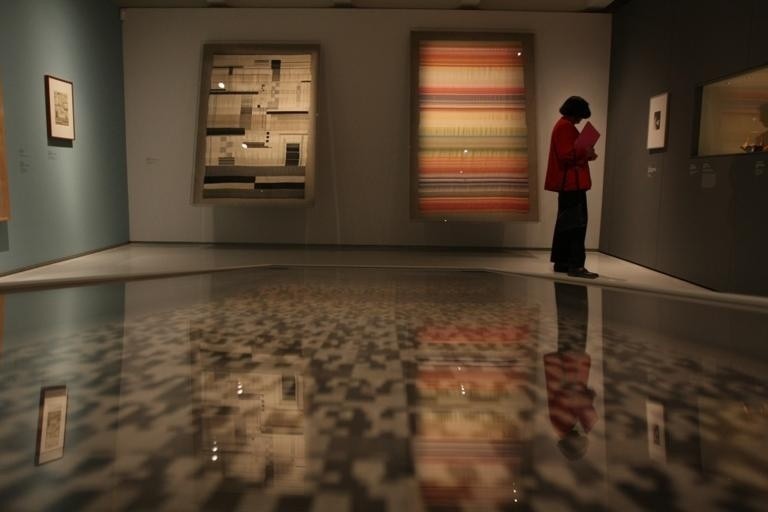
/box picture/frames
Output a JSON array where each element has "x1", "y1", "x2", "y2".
[
  {"x1": 33, "y1": 383, "x2": 70, "y2": 466},
  {"x1": 644, "y1": 398, "x2": 669, "y2": 460},
  {"x1": 188, "y1": 39, "x2": 323, "y2": 208},
  {"x1": 44, "y1": 74, "x2": 76, "y2": 142},
  {"x1": 406, "y1": 28, "x2": 541, "y2": 226},
  {"x1": 645, "y1": 91, "x2": 673, "y2": 154}
]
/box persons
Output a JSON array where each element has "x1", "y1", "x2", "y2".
[
  {"x1": 542, "y1": 94, "x2": 599, "y2": 280},
  {"x1": 543, "y1": 274, "x2": 598, "y2": 463}
]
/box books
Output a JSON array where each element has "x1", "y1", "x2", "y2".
[{"x1": 575, "y1": 121, "x2": 601, "y2": 148}]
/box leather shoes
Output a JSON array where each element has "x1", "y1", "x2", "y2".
[
  {"x1": 554, "y1": 262, "x2": 568, "y2": 273},
  {"x1": 568, "y1": 265, "x2": 599, "y2": 279}
]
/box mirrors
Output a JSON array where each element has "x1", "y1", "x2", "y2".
[{"x1": 691, "y1": 65, "x2": 767, "y2": 159}]
